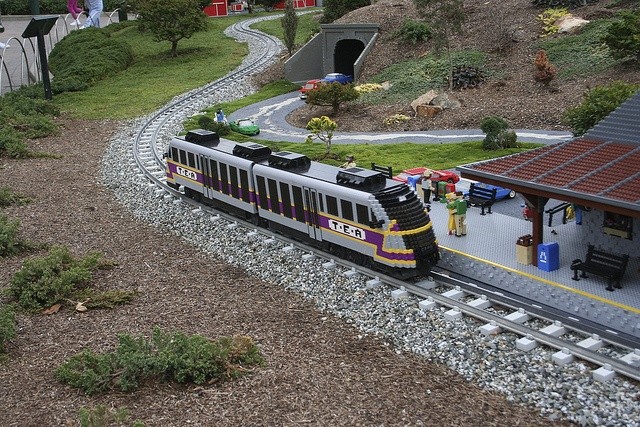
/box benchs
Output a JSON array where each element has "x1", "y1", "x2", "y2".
[
  {"x1": 570, "y1": 244, "x2": 630, "y2": 292},
  {"x1": 462, "y1": 182, "x2": 498, "y2": 215}
]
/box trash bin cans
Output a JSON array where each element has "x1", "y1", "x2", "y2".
[
  {"x1": 537, "y1": 241, "x2": 559, "y2": 272},
  {"x1": 516, "y1": 234, "x2": 533, "y2": 265}
]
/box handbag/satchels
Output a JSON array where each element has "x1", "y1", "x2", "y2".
[{"x1": 84, "y1": 9, "x2": 89, "y2": 17}]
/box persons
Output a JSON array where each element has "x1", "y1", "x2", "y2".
[
  {"x1": 66, "y1": 0, "x2": 84, "y2": 26},
  {"x1": 80, "y1": 0, "x2": 103, "y2": 30}
]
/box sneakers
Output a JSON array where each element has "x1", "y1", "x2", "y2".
[{"x1": 70, "y1": 19, "x2": 81, "y2": 26}]
